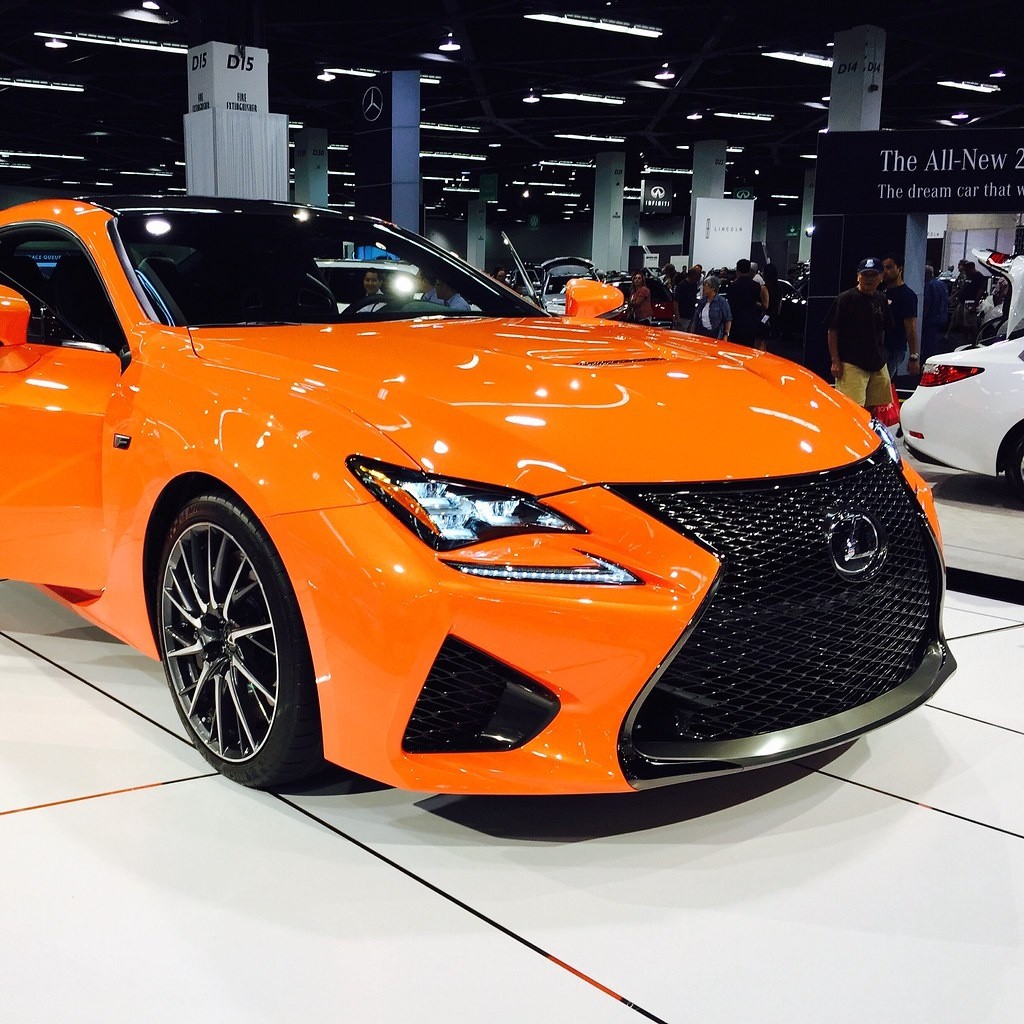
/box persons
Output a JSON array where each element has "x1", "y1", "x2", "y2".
[
  {"x1": 414, "y1": 266, "x2": 444, "y2": 306},
  {"x1": 920, "y1": 265, "x2": 949, "y2": 358},
  {"x1": 512, "y1": 283, "x2": 522, "y2": 295},
  {"x1": 433, "y1": 279, "x2": 472, "y2": 312},
  {"x1": 945, "y1": 259, "x2": 970, "y2": 339},
  {"x1": 361, "y1": 265, "x2": 389, "y2": 312},
  {"x1": 947, "y1": 265, "x2": 958, "y2": 277},
  {"x1": 879, "y1": 253, "x2": 921, "y2": 382},
  {"x1": 958, "y1": 262, "x2": 986, "y2": 339},
  {"x1": 595, "y1": 259, "x2": 784, "y2": 351},
  {"x1": 822, "y1": 257, "x2": 897, "y2": 413},
  {"x1": 496, "y1": 270, "x2": 509, "y2": 287}
]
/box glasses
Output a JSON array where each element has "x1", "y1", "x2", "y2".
[
  {"x1": 702, "y1": 282, "x2": 711, "y2": 287},
  {"x1": 634, "y1": 276, "x2": 642, "y2": 279}
]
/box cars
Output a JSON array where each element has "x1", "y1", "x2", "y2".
[
  {"x1": 308, "y1": 255, "x2": 483, "y2": 322},
  {"x1": 599, "y1": 273, "x2": 681, "y2": 332},
  {"x1": 896, "y1": 247, "x2": 1024, "y2": 509},
  {"x1": 539, "y1": 257, "x2": 603, "y2": 320},
  {"x1": 0, "y1": 191, "x2": 958, "y2": 800}
]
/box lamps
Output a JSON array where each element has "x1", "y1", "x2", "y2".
[{"x1": 0, "y1": 1, "x2": 1004, "y2": 215}]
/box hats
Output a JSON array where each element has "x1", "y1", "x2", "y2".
[{"x1": 857, "y1": 256, "x2": 884, "y2": 274}]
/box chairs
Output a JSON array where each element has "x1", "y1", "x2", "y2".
[
  {"x1": 138, "y1": 254, "x2": 176, "y2": 286},
  {"x1": 47, "y1": 251, "x2": 141, "y2": 354},
  {"x1": 2, "y1": 259, "x2": 51, "y2": 317},
  {"x1": 212, "y1": 251, "x2": 338, "y2": 319}
]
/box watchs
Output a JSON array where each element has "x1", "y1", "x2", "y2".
[{"x1": 909, "y1": 352, "x2": 919, "y2": 361}]
